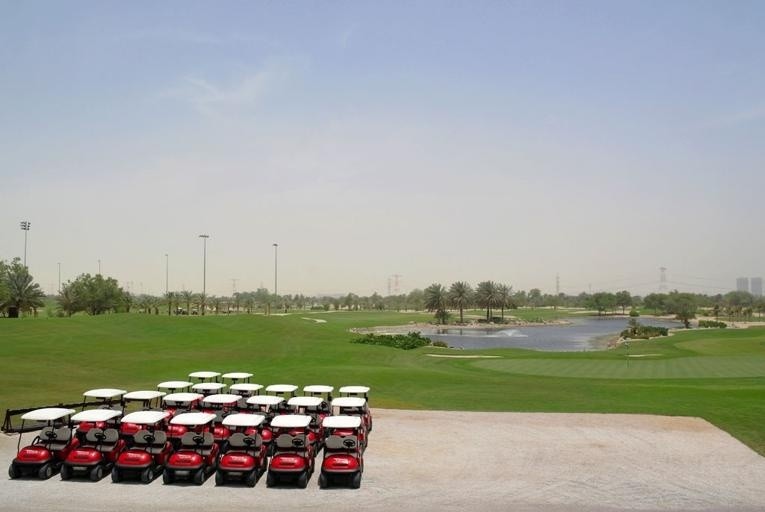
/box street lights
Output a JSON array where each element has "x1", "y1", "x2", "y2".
[
  {"x1": 19, "y1": 219, "x2": 32, "y2": 266},
  {"x1": 199, "y1": 233, "x2": 209, "y2": 294},
  {"x1": 272, "y1": 243, "x2": 278, "y2": 295}
]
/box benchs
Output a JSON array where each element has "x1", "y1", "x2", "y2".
[{"x1": 32, "y1": 402, "x2": 365, "y2": 458}]
